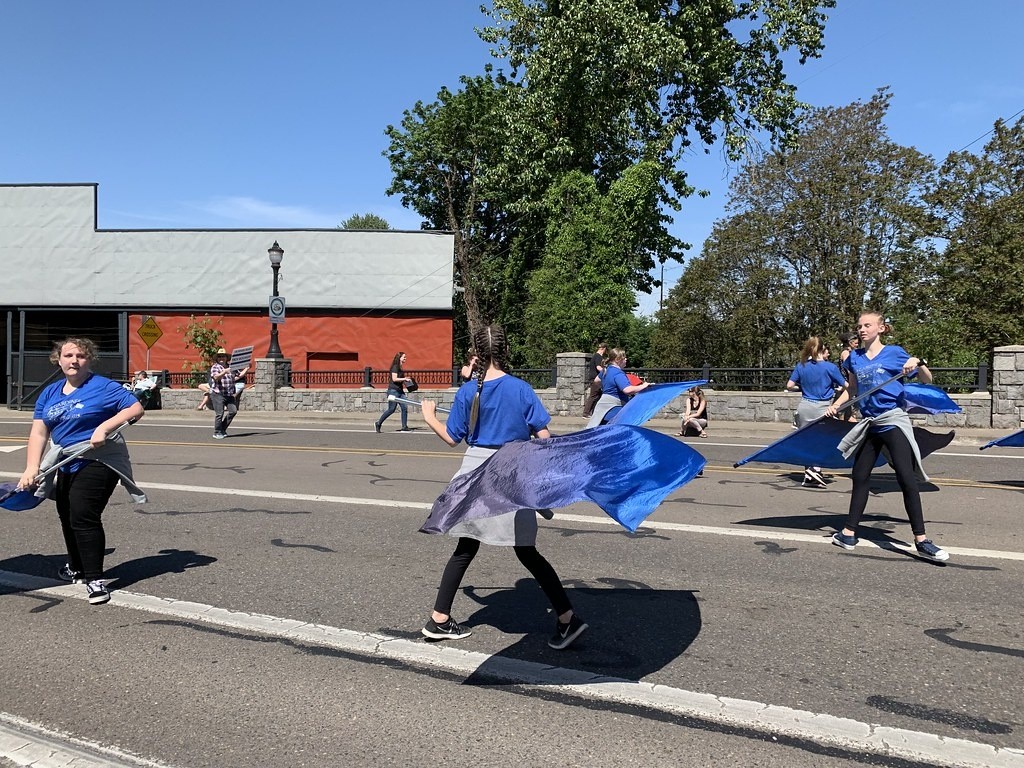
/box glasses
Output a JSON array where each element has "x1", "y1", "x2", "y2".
[
  {"x1": 689, "y1": 391, "x2": 693, "y2": 393},
  {"x1": 217, "y1": 356, "x2": 226, "y2": 358}
]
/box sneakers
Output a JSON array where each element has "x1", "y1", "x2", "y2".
[
  {"x1": 548, "y1": 614, "x2": 588, "y2": 649},
  {"x1": 374, "y1": 422, "x2": 381, "y2": 433},
  {"x1": 86, "y1": 579, "x2": 110, "y2": 603},
  {"x1": 58, "y1": 563, "x2": 87, "y2": 584},
  {"x1": 914, "y1": 539, "x2": 949, "y2": 561},
  {"x1": 213, "y1": 432, "x2": 228, "y2": 439},
  {"x1": 833, "y1": 530, "x2": 855, "y2": 550},
  {"x1": 802, "y1": 478, "x2": 827, "y2": 489},
  {"x1": 805, "y1": 466, "x2": 827, "y2": 486},
  {"x1": 421, "y1": 614, "x2": 472, "y2": 640}
]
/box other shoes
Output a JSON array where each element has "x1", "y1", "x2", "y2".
[
  {"x1": 701, "y1": 433, "x2": 707, "y2": 438},
  {"x1": 401, "y1": 427, "x2": 413, "y2": 432},
  {"x1": 582, "y1": 415, "x2": 591, "y2": 420},
  {"x1": 674, "y1": 432, "x2": 681, "y2": 436}
]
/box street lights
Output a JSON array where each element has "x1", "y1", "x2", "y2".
[{"x1": 265, "y1": 241, "x2": 286, "y2": 358}]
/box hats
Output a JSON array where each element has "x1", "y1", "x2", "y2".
[
  {"x1": 598, "y1": 343, "x2": 608, "y2": 351},
  {"x1": 213, "y1": 349, "x2": 231, "y2": 363}
]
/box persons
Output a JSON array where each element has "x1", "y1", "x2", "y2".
[
  {"x1": 421, "y1": 326, "x2": 588, "y2": 650},
  {"x1": 821, "y1": 333, "x2": 858, "y2": 422},
  {"x1": 17, "y1": 339, "x2": 145, "y2": 604},
  {"x1": 197, "y1": 358, "x2": 247, "y2": 410},
  {"x1": 461, "y1": 348, "x2": 483, "y2": 387},
  {"x1": 825, "y1": 311, "x2": 950, "y2": 561},
  {"x1": 674, "y1": 386, "x2": 708, "y2": 438},
  {"x1": 211, "y1": 349, "x2": 250, "y2": 439},
  {"x1": 786, "y1": 337, "x2": 849, "y2": 488},
  {"x1": 593, "y1": 348, "x2": 650, "y2": 430},
  {"x1": 374, "y1": 352, "x2": 413, "y2": 433},
  {"x1": 582, "y1": 343, "x2": 611, "y2": 419},
  {"x1": 131, "y1": 371, "x2": 156, "y2": 400}
]
